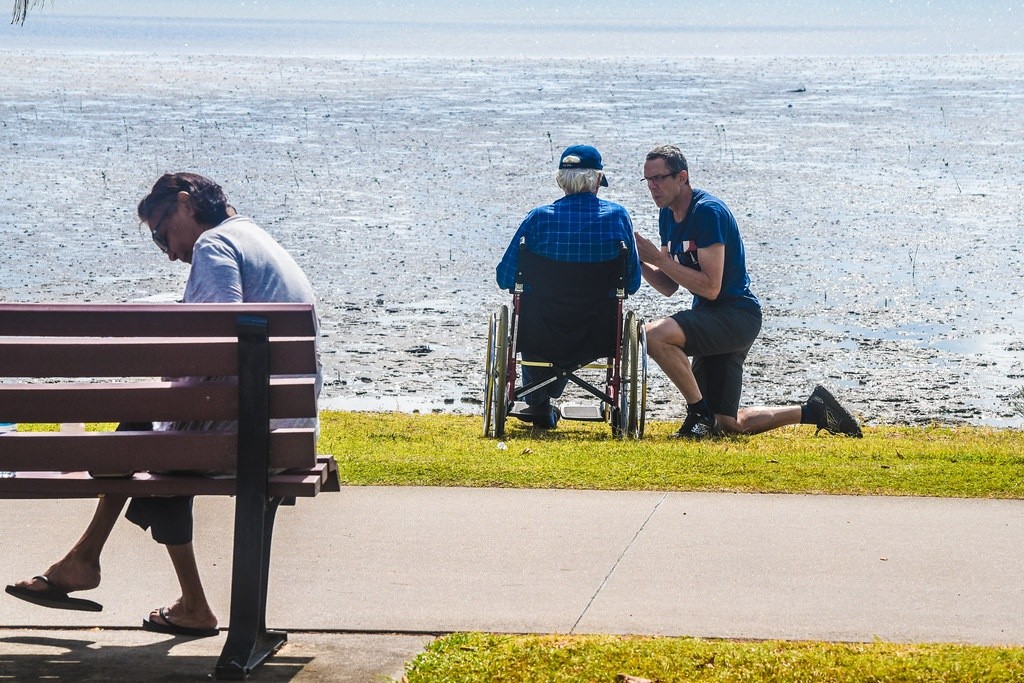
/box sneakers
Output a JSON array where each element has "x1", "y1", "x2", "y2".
[
  {"x1": 806, "y1": 383, "x2": 863, "y2": 440},
  {"x1": 668, "y1": 404, "x2": 717, "y2": 442}
]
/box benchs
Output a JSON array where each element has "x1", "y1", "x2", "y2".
[{"x1": 0, "y1": 301, "x2": 345, "y2": 683}]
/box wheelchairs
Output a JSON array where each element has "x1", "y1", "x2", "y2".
[{"x1": 481, "y1": 264, "x2": 651, "y2": 441}]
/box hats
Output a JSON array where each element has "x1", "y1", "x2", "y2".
[{"x1": 559, "y1": 144, "x2": 608, "y2": 187}]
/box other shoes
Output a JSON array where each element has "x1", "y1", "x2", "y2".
[{"x1": 517, "y1": 405, "x2": 561, "y2": 430}]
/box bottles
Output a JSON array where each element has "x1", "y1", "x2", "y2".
[
  {"x1": 0, "y1": 423, "x2": 17, "y2": 479},
  {"x1": 60, "y1": 423, "x2": 85, "y2": 433}
]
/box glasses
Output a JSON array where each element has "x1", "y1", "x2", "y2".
[
  {"x1": 152, "y1": 205, "x2": 173, "y2": 251},
  {"x1": 640, "y1": 171, "x2": 679, "y2": 188}
]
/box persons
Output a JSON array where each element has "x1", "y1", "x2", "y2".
[
  {"x1": 633, "y1": 144, "x2": 863, "y2": 444},
  {"x1": 496, "y1": 144, "x2": 642, "y2": 429},
  {"x1": 4, "y1": 171, "x2": 322, "y2": 634}
]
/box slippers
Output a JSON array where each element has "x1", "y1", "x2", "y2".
[
  {"x1": 5, "y1": 575, "x2": 103, "y2": 613},
  {"x1": 142, "y1": 607, "x2": 220, "y2": 636}
]
